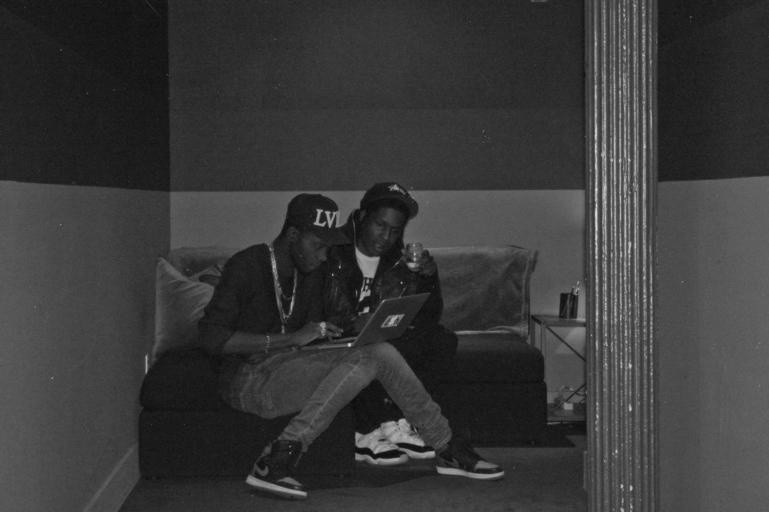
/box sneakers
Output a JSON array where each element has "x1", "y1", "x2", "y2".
[
  {"x1": 381, "y1": 418, "x2": 436, "y2": 459},
  {"x1": 353, "y1": 428, "x2": 408, "y2": 466},
  {"x1": 246, "y1": 441, "x2": 308, "y2": 499},
  {"x1": 436, "y1": 440, "x2": 505, "y2": 479}
]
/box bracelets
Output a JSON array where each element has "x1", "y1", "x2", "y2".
[{"x1": 261, "y1": 331, "x2": 272, "y2": 354}]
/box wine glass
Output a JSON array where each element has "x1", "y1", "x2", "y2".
[{"x1": 406, "y1": 243, "x2": 425, "y2": 288}]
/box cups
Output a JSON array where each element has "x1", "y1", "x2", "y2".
[{"x1": 558, "y1": 293, "x2": 578, "y2": 320}]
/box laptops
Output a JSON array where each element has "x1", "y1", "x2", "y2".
[{"x1": 299, "y1": 293, "x2": 430, "y2": 351}]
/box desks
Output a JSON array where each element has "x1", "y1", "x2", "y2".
[{"x1": 532, "y1": 314, "x2": 594, "y2": 445}]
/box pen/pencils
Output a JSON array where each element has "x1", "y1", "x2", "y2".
[{"x1": 560, "y1": 280, "x2": 580, "y2": 320}]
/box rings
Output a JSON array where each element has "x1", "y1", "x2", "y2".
[
  {"x1": 320, "y1": 328, "x2": 326, "y2": 337},
  {"x1": 319, "y1": 321, "x2": 326, "y2": 328}
]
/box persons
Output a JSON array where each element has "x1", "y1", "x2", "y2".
[
  {"x1": 193, "y1": 193, "x2": 506, "y2": 502},
  {"x1": 324, "y1": 181, "x2": 459, "y2": 465}
]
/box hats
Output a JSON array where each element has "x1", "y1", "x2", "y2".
[
  {"x1": 287, "y1": 194, "x2": 351, "y2": 246},
  {"x1": 360, "y1": 182, "x2": 419, "y2": 219}
]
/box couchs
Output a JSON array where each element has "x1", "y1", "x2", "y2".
[{"x1": 137, "y1": 243, "x2": 547, "y2": 480}]
